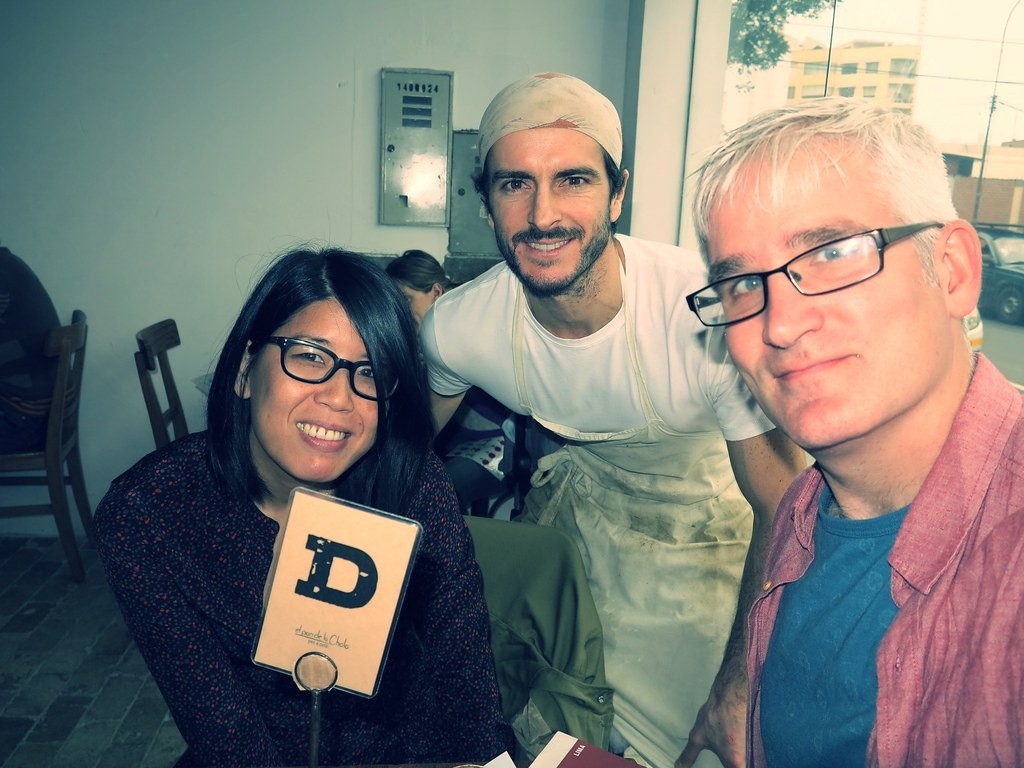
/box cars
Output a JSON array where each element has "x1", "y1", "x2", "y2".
[{"x1": 973, "y1": 227, "x2": 1024, "y2": 325}]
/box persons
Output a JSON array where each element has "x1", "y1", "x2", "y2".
[
  {"x1": 385, "y1": 248, "x2": 514, "y2": 515},
  {"x1": 81, "y1": 251, "x2": 507, "y2": 768},
  {"x1": 688, "y1": 93, "x2": 1023, "y2": 768},
  {"x1": 420, "y1": 70, "x2": 809, "y2": 768},
  {"x1": 0, "y1": 243, "x2": 77, "y2": 457}
]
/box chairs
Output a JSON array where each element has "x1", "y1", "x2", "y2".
[
  {"x1": 0, "y1": 309, "x2": 93, "y2": 586},
  {"x1": 462, "y1": 516, "x2": 615, "y2": 768},
  {"x1": 134, "y1": 319, "x2": 189, "y2": 449}
]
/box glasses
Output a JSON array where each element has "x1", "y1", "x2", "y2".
[
  {"x1": 686, "y1": 220, "x2": 946, "y2": 327},
  {"x1": 264, "y1": 336, "x2": 400, "y2": 401}
]
